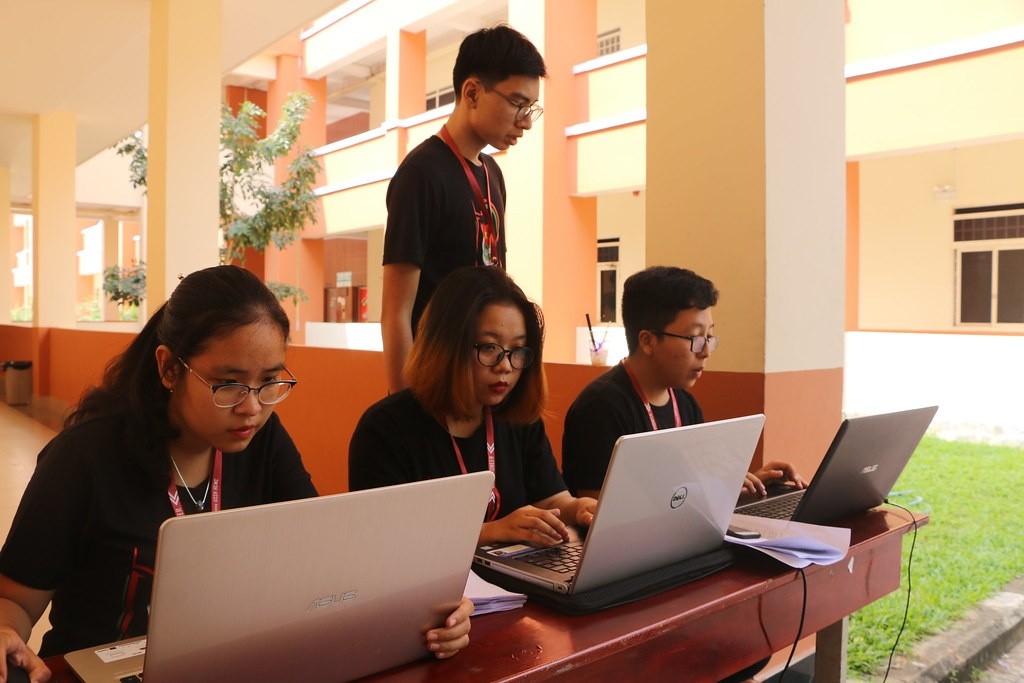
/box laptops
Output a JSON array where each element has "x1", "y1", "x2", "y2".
[
  {"x1": 63, "y1": 470, "x2": 496, "y2": 683},
  {"x1": 474, "y1": 413, "x2": 766, "y2": 594},
  {"x1": 733, "y1": 406, "x2": 939, "y2": 525}
]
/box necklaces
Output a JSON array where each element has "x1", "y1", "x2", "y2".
[{"x1": 171, "y1": 457, "x2": 210, "y2": 511}]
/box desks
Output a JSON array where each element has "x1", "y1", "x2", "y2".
[{"x1": 49, "y1": 502, "x2": 931, "y2": 683}]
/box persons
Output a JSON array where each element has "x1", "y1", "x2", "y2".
[
  {"x1": 0, "y1": 264, "x2": 474, "y2": 683},
  {"x1": 382, "y1": 26, "x2": 547, "y2": 395},
  {"x1": 561, "y1": 265, "x2": 808, "y2": 499},
  {"x1": 348, "y1": 266, "x2": 598, "y2": 548}
]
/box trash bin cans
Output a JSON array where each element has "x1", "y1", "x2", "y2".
[{"x1": 3, "y1": 361, "x2": 33, "y2": 404}]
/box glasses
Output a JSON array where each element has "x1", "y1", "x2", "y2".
[
  {"x1": 473, "y1": 342, "x2": 535, "y2": 370},
  {"x1": 651, "y1": 329, "x2": 719, "y2": 353},
  {"x1": 174, "y1": 352, "x2": 298, "y2": 408},
  {"x1": 484, "y1": 83, "x2": 544, "y2": 122}
]
